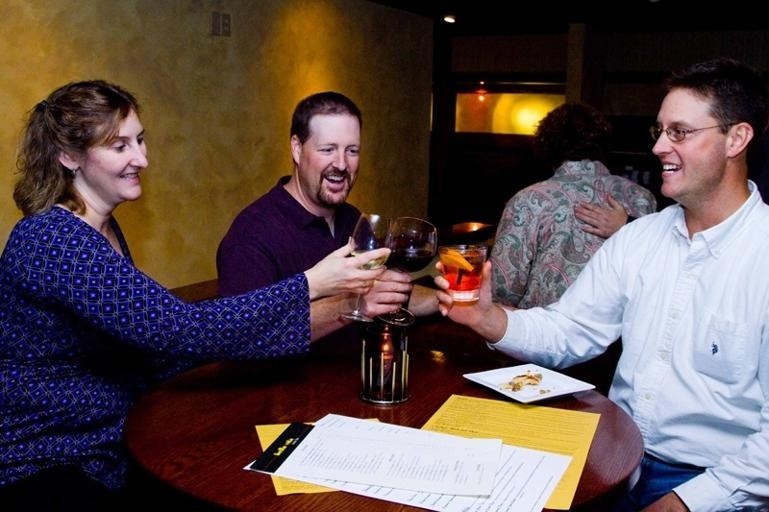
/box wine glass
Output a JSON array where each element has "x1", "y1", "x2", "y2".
[
  {"x1": 375, "y1": 216, "x2": 438, "y2": 326},
  {"x1": 341, "y1": 212, "x2": 393, "y2": 323}
]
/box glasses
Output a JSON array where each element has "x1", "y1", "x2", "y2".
[{"x1": 652, "y1": 121, "x2": 739, "y2": 141}]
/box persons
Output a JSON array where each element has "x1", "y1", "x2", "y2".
[
  {"x1": 215, "y1": 91, "x2": 441, "y2": 342},
  {"x1": 486, "y1": 100, "x2": 658, "y2": 400},
  {"x1": 0, "y1": 79, "x2": 393, "y2": 512},
  {"x1": 433, "y1": 55, "x2": 769, "y2": 511},
  {"x1": 575, "y1": 195, "x2": 639, "y2": 242}
]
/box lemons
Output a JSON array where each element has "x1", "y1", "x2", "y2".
[{"x1": 440, "y1": 249, "x2": 473, "y2": 271}]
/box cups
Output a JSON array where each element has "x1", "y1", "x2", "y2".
[{"x1": 439, "y1": 245, "x2": 487, "y2": 307}]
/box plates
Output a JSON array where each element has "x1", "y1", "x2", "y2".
[{"x1": 462, "y1": 361, "x2": 598, "y2": 405}]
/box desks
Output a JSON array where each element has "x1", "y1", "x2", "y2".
[{"x1": 119, "y1": 306, "x2": 654, "y2": 511}]
